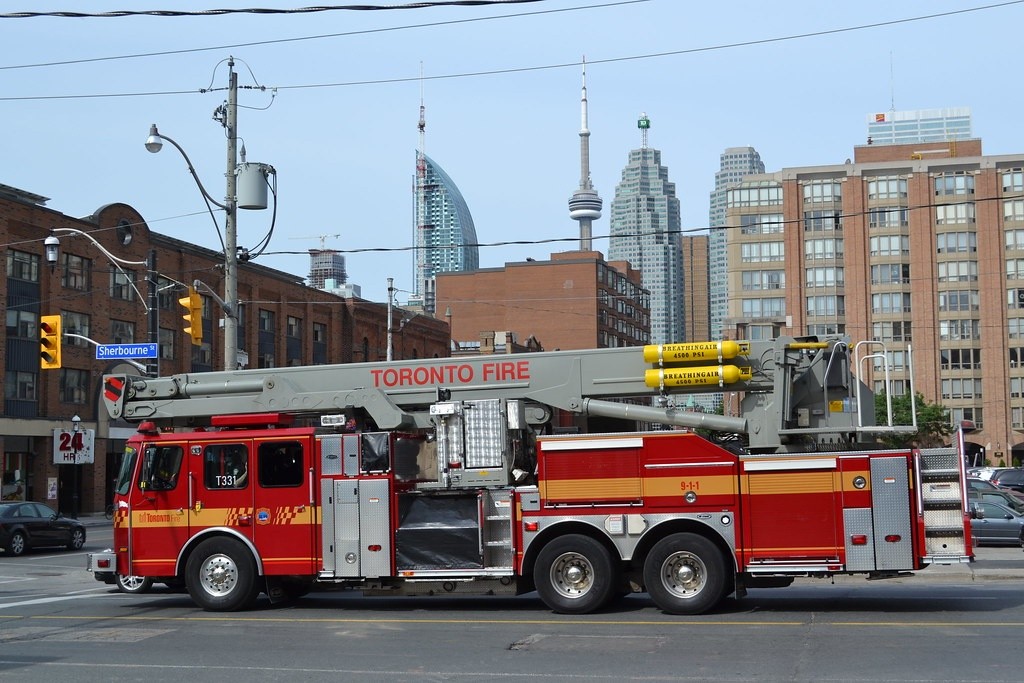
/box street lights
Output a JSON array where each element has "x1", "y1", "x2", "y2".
[
  {"x1": 143, "y1": 123, "x2": 238, "y2": 372},
  {"x1": 43, "y1": 226, "x2": 160, "y2": 377}
]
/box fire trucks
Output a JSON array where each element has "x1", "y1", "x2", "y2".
[{"x1": 88, "y1": 333, "x2": 977, "y2": 618}]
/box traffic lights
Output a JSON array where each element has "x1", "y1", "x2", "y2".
[
  {"x1": 179, "y1": 285, "x2": 203, "y2": 347},
  {"x1": 40, "y1": 315, "x2": 61, "y2": 369}
]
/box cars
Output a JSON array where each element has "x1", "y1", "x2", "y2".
[
  {"x1": 0, "y1": 500, "x2": 87, "y2": 557},
  {"x1": 965, "y1": 465, "x2": 1024, "y2": 548}
]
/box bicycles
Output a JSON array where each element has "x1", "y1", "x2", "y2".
[{"x1": 104, "y1": 505, "x2": 115, "y2": 519}]
